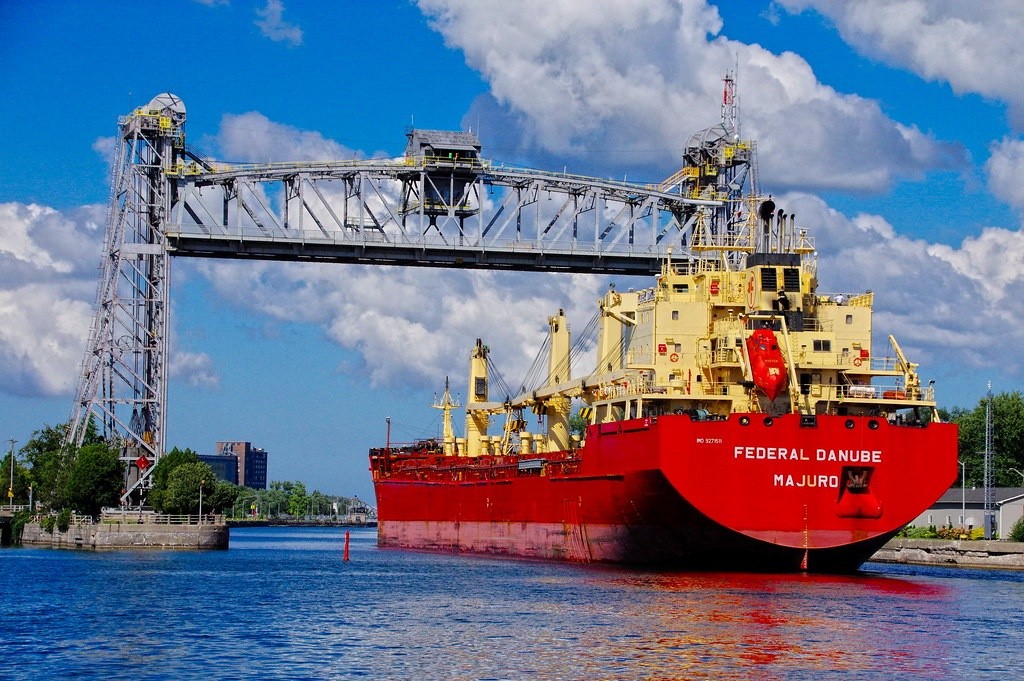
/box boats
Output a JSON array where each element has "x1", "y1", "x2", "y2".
[{"x1": 368, "y1": 196, "x2": 960, "y2": 573}]
